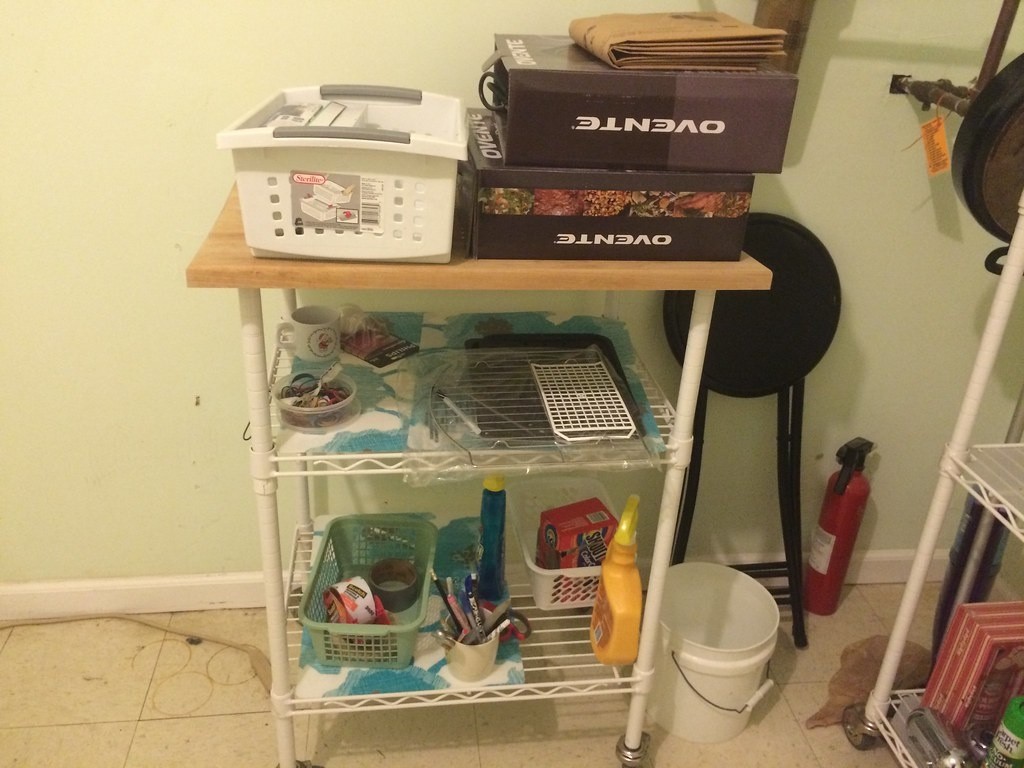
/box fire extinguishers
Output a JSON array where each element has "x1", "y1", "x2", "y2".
[{"x1": 802, "y1": 436, "x2": 874, "y2": 616}]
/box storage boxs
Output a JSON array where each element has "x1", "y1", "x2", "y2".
[
  {"x1": 636, "y1": 561, "x2": 781, "y2": 746},
  {"x1": 479, "y1": 32, "x2": 800, "y2": 174},
  {"x1": 212, "y1": 80, "x2": 470, "y2": 267},
  {"x1": 457, "y1": 106, "x2": 756, "y2": 263}
]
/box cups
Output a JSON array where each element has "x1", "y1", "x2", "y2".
[
  {"x1": 274, "y1": 306, "x2": 341, "y2": 361},
  {"x1": 442, "y1": 608, "x2": 498, "y2": 684}
]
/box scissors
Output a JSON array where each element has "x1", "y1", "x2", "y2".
[{"x1": 478, "y1": 598, "x2": 531, "y2": 642}]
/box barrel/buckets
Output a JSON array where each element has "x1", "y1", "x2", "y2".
[{"x1": 640, "y1": 561, "x2": 780, "y2": 743}]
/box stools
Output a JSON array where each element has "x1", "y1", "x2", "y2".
[{"x1": 664, "y1": 210, "x2": 843, "y2": 653}]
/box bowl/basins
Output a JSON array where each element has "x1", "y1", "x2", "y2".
[{"x1": 270, "y1": 370, "x2": 357, "y2": 430}]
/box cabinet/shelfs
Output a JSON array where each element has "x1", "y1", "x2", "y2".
[
  {"x1": 180, "y1": 181, "x2": 777, "y2": 768},
  {"x1": 847, "y1": 179, "x2": 1024, "y2": 768}
]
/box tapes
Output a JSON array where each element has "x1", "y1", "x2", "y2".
[
  {"x1": 367, "y1": 558, "x2": 418, "y2": 612},
  {"x1": 329, "y1": 575, "x2": 376, "y2": 624}
]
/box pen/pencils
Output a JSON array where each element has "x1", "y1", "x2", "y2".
[
  {"x1": 436, "y1": 389, "x2": 481, "y2": 435},
  {"x1": 429, "y1": 568, "x2": 511, "y2": 644}
]
